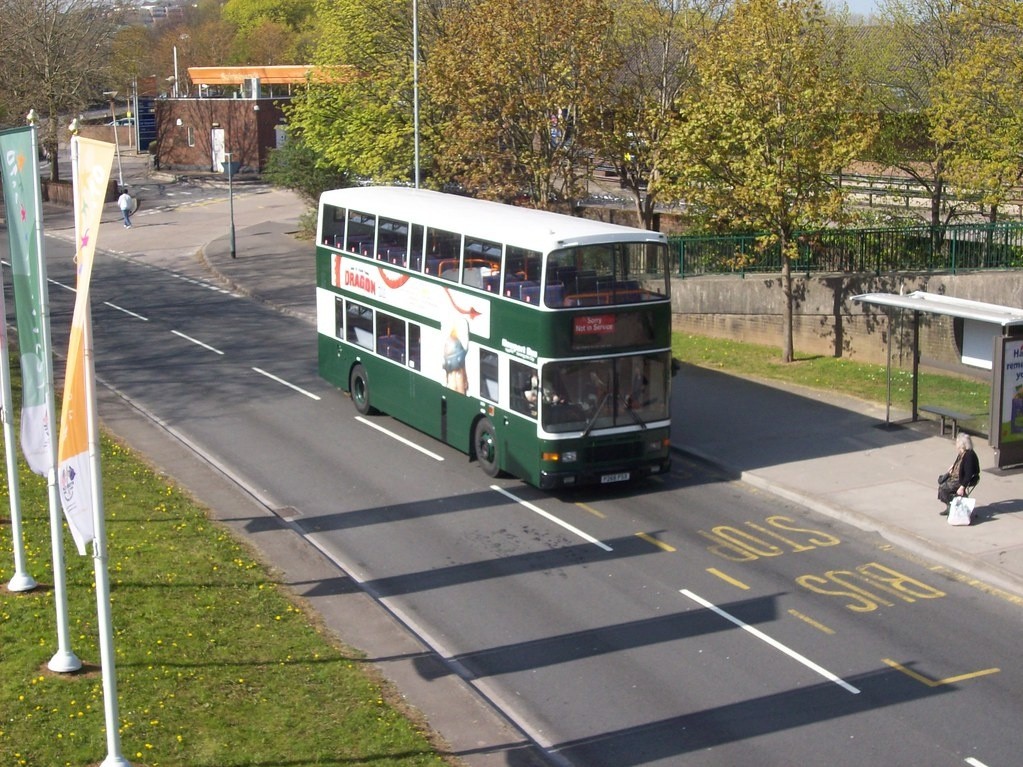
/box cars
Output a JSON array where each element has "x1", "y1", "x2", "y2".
[{"x1": 106, "y1": 118, "x2": 134, "y2": 127}]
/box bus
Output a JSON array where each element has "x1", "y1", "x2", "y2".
[{"x1": 315, "y1": 187, "x2": 680, "y2": 492}]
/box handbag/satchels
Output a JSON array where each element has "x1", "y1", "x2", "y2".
[
  {"x1": 937, "y1": 453, "x2": 961, "y2": 485},
  {"x1": 946, "y1": 496, "x2": 975, "y2": 526}
]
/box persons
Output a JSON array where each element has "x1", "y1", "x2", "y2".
[
  {"x1": 443, "y1": 336, "x2": 468, "y2": 395},
  {"x1": 937, "y1": 432, "x2": 980, "y2": 516},
  {"x1": 118, "y1": 189, "x2": 132, "y2": 229},
  {"x1": 524, "y1": 370, "x2": 564, "y2": 414},
  {"x1": 154, "y1": 154, "x2": 160, "y2": 170}
]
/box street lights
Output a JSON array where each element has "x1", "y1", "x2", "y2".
[{"x1": 224, "y1": 153, "x2": 235, "y2": 257}]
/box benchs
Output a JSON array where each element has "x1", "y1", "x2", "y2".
[{"x1": 918, "y1": 403, "x2": 977, "y2": 439}]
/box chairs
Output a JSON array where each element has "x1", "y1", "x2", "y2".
[
  {"x1": 947, "y1": 471, "x2": 981, "y2": 518},
  {"x1": 378, "y1": 334, "x2": 644, "y2": 421},
  {"x1": 335, "y1": 232, "x2": 643, "y2": 304}
]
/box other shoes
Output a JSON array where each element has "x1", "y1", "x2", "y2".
[
  {"x1": 124, "y1": 225, "x2": 126, "y2": 228},
  {"x1": 127, "y1": 225, "x2": 131, "y2": 229},
  {"x1": 940, "y1": 509, "x2": 948, "y2": 515}
]
